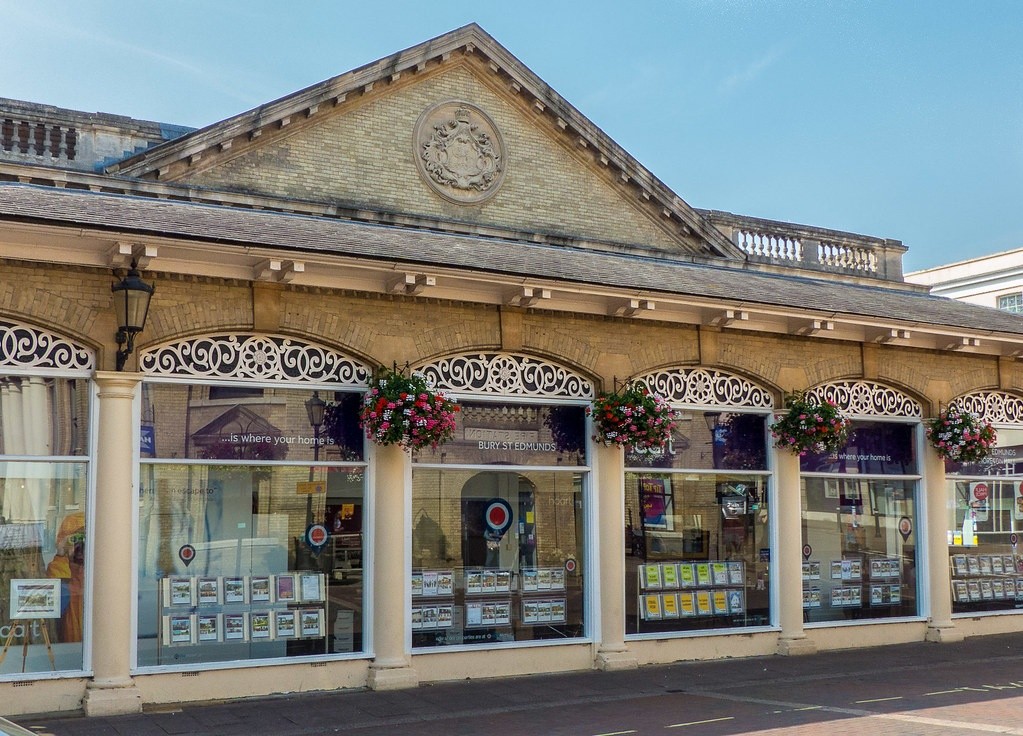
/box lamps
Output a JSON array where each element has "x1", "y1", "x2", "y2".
[{"x1": 111, "y1": 257, "x2": 156, "y2": 372}]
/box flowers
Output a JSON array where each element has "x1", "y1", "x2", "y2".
[
  {"x1": 864, "y1": 421, "x2": 913, "y2": 467},
  {"x1": 927, "y1": 408, "x2": 998, "y2": 465},
  {"x1": 358, "y1": 364, "x2": 463, "y2": 456},
  {"x1": 583, "y1": 380, "x2": 682, "y2": 457},
  {"x1": 767, "y1": 389, "x2": 857, "y2": 457},
  {"x1": 321, "y1": 392, "x2": 364, "y2": 462},
  {"x1": 719, "y1": 412, "x2": 767, "y2": 471},
  {"x1": 542, "y1": 405, "x2": 585, "y2": 463}
]
